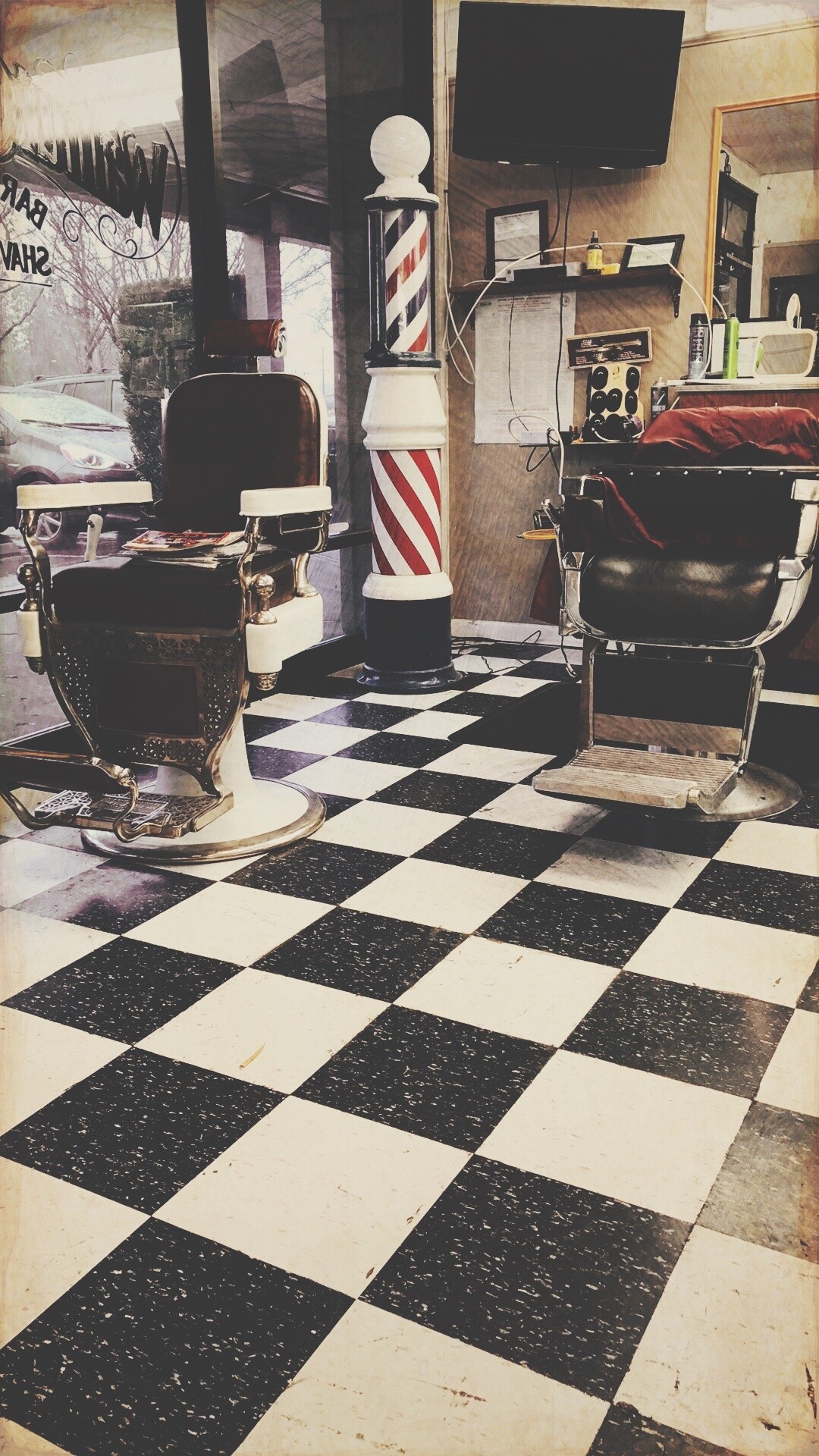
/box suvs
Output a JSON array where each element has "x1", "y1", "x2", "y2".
[{"x1": 0, "y1": 368, "x2": 147, "y2": 548}]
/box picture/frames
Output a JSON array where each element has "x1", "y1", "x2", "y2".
[
  {"x1": 484, "y1": 197, "x2": 550, "y2": 281},
  {"x1": 618, "y1": 232, "x2": 688, "y2": 273}
]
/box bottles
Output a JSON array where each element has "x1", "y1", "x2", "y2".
[
  {"x1": 584, "y1": 230, "x2": 604, "y2": 276},
  {"x1": 650, "y1": 376, "x2": 669, "y2": 427},
  {"x1": 724, "y1": 312, "x2": 740, "y2": 378}
]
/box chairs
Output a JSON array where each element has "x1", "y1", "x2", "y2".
[
  {"x1": 531, "y1": 462, "x2": 819, "y2": 829},
  {"x1": 10, "y1": 366, "x2": 337, "y2": 868}
]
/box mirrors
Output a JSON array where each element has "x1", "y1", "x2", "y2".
[{"x1": 698, "y1": 87, "x2": 818, "y2": 378}]
